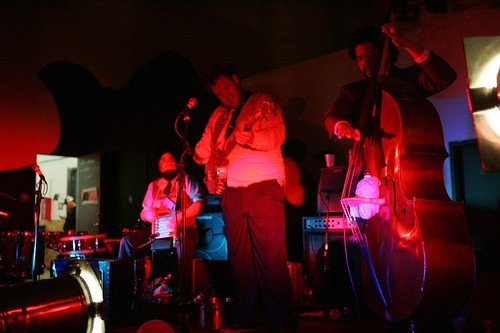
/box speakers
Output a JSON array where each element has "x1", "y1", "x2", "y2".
[
  {"x1": 303, "y1": 230, "x2": 368, "y2": 321},
  {"x1": 317, "y1": 173, "x2": 346, "y2": 213}
]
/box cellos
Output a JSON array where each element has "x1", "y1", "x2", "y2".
[{"x1": 334, "y1": 2, "x2": 477, "y2": 327}]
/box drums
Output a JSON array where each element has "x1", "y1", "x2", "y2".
[
  {"x1": 57, "y1": 232, "x2": 105, "y2": 256},
  {"x1": 3, "y1": 230, "x2": 35, "y2": 266}
]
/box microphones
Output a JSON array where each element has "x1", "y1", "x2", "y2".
[
  {"x1": 149, "y1": 233, "x2": 160, "y2": 239},
  {"x1": 320, "y1": 189, "x2": 335, "y2": 193},
  {"x1": 177, "y1": 98, "x2": 197, "y2": 122},
  {"x1": 32, "y1": 164, "x2": 47, "y2": 185}
]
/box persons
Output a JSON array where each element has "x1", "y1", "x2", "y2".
[
  {"x1": 118, "y1": 152, "x2": 205, "y2": 305},
  {"x1": 325, "y1": 18, "x2": 457, "y2": 142},
  {"x1": 193, "y1": 66, "x2": 298, "y2": 333},
  {"x1": 59, "y1": 195, "x2": 76, "y2": 231},
  {"x1": 280, "y1": 139, "x2": 311, "y2": 315}
]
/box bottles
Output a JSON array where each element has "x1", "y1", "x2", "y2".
[{"x1": 211, "y1": 297, "x2": 223, "y2": 331}]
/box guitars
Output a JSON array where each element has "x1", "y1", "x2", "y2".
[{"x1": 204, "y1": 95, "x2": 277, "y2": 194}]
